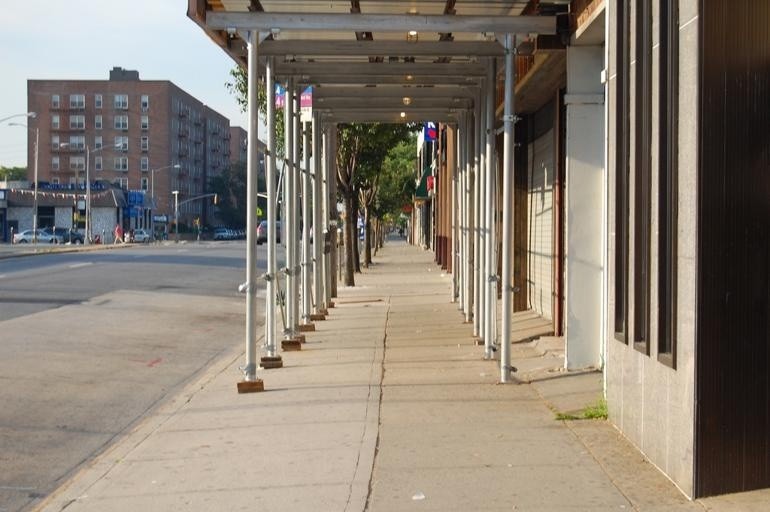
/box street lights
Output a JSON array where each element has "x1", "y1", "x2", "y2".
[
  {"x1": 86, "y1": 142, "x2": 122, "y2": 244},
  {"x1": 151, "y1": 164, "x2": 181, "y2": 199},
  {"x1": 10, "y1": 121, "x2": 39, "y2": 243},
  {"x1": 0, "y1": 111, "x2": 38, "y2": 125},
  {"x1": 60, "y1": 142, "x2": 90, "y2": 247}
]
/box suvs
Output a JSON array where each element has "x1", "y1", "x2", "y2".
[
  {"x1": 40, "y1": 227, "x2": 83, "y2": 246},
  {"x1": 257, "y1": 221, "x2": 280, "y2": 246}
]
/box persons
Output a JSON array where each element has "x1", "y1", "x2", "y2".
[
  {"x1": 128, "y1": 228, "x2": 135, "y2": 243},
  {"x1": 113, "y1": 224, "x2": 126, "y2": 244}
]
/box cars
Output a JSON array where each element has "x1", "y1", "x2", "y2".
[
  {"x1": 133, "y1": 229, "x2": 149, "y2": 242},
  {"x1": 214, "y1": 228, "x2": 246, "y2": 239},
  {"x1": 309, "y1": 226, "x2": 313, "y2": 243},
  {"x1": 13, "y1": 230, "x2": 65, "y2": 246}
]
